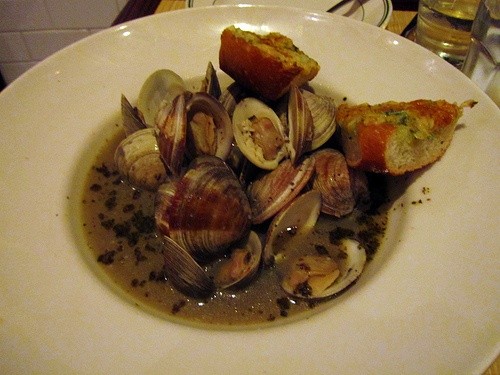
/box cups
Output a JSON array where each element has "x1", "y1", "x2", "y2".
[
  {"x1": 460, "y1": 1, "x2": 499, "y2": 107},
  {"x1": 416, "y1": 0, "x2": 482, "y2": 71}
]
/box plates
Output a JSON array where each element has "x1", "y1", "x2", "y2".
[
  {"x1": 0, "y1": 5, "x2": 499, "y2": 375},
  {"x1": 185, "y1": 0, "x2": 393, "y2": 31}
]
